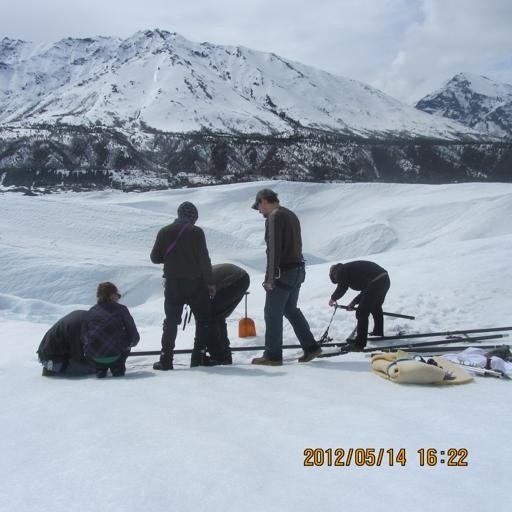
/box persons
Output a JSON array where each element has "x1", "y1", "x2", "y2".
[
  {"x1": 148, "y1": 201, "x2": 218, "y2": 370},
  {"x1": 36, "y1": 308, "x2": 96, "y2": 376},
  {"x1": 251, "y1": 189, "x2": 322, "y2": 365},
  {"x1": 328, "y1": 261, "x2": 391, "y2": 351},
  {"x1": 80, "y1": 282, "x2": 140, "y2": 378},
  {"x1": 191, "y1": 264, "x2": 250, "y2": 367}
]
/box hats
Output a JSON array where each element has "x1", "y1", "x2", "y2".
[
  {"x1": 329, "y1": 263, "x2": 343, "y2": 284},
  {"x1": 252, "y1": 189, "x2": 275, "y2": 209}
]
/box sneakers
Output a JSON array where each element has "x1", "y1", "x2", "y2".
[
  {"x1": 370, "y1": 332, "x2": 383, "y2": 336},
  {"x1": 191, "y1": 345, "x2": 232, "y2": 366},
  {"x1": 342, "y1": 343, "x2": 364, "y2": 352},
  {"x1": 110, "y1": 368, "x2": 124, "y2": 376},
  {"x1": 252, "y1": 357, "x2": 282, "y2": 367},
  {"x1": 298, "y1": 347, "x2": 322, "y2": 362},
  {"x1": 97, "y1": 368, "x2": 107, "y2": 378}
]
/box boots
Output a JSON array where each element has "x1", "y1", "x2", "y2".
[{"x1": 153, "y1": 349, "x2": 174, "y2": 370}]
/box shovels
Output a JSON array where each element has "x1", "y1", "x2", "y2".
[{"x1": 239, "y1": 292, "x2": 256, "y2": 337}]
